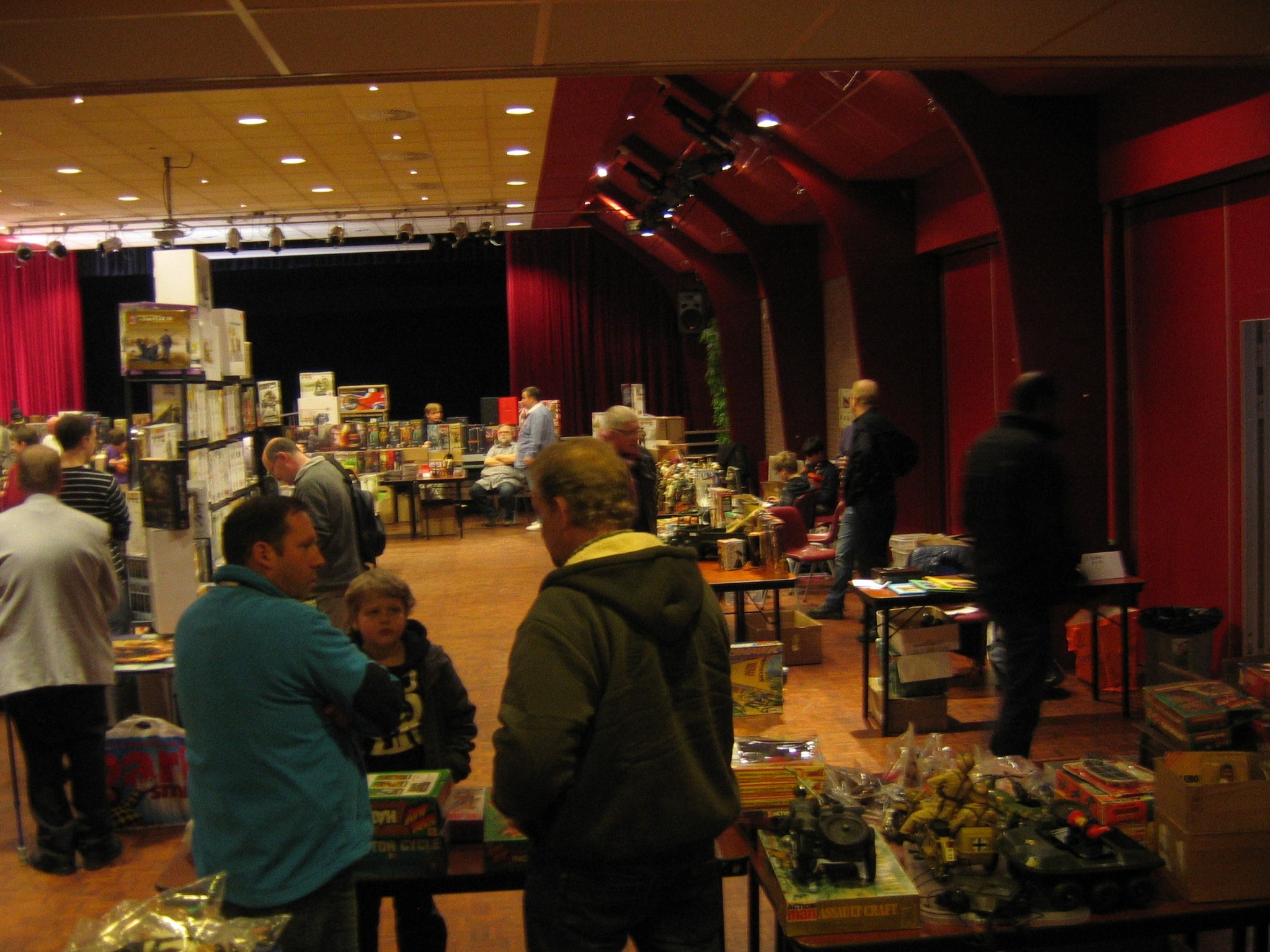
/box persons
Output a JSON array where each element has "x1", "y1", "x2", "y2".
[
  {"x1": 342, "y1": 568, "x2": 479, "y2": 952},
  {"x1": 261, "y1": 437, "x2": 367, "y2": 630},
  {"x1": 783, "y1": 784, "x2": 820, "y2": 884},
  {"x1": 94, "y1": 417, "x2": 109, "y2": 444},
  {"x1": 887, "y1": 754, "x2": 990, "y2": 845},
  {"x1": 765, "y1": 450, "x2": 817, "y2": 530},
  {"x1": 469, "y1": 423, "x2": 523, "y2": 527},
  {"x1": 960, "y1": 371, "x2": 1080, "y2": 755},
  {"x1": 0, "y1": 427, "x2": 44, "y2": 513},
  {"x1": 54, "y1": 414, "x2": 132, "y2": 636},
  {"x1": 173, "y1": 493, "x2": 400, "y2": 952},
  {"x1": 421, "y1": 402, "x2": 444, "y2": 442},
  {"x1": 515, "y1": 386, "x2": 557, "y2": 531},
  {"x1": 490, "y1": 436, "x2": 737, "y2": 952},
  {"x1": 0, "y1": 445, "x2": 126, "y2": 875},
  {"x1": 5, "y1": 409, "x2": 27, "y2": 432},
  {"x1": 799, "y1": 436, "x2": 840, "y2": 516},
  {"x1": 805, "y1": 378, "x2": 903, "y2": 642},
  {"x1": 599, "y1": 405, "x2": 659, "y2": 536},
  {"x1": 160, "y1": 329, "x2": 172, "y2": 363},
  {"x1": 655, "y1": 455, "x2": 736, "y2": 515}
]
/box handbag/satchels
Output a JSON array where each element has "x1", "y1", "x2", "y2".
[{"x1": 81, "y1": 712, "x2": 200, "y2": 833}]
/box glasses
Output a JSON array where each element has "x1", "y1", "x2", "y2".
[
  {"x1": 773, "y1": 467, "x2": 781, "y2": 478},
  {"x1": 266, "y1": 461, "x2": 277, "y2": 478},
  {"x1": 612, "y1": 425, "x2": 644, "y2": 434}
]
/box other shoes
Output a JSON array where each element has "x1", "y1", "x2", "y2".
[
  {"x1": 484, "y1": 517, "x2": 497, "y2": 527},
  {"x1": 503, "y1": 518, "x2": 512, "y2": 526},
  {"x1": 78, "y1": 833, "x2": 124, "y2": 872},
  {"x1": 858, "y1": 606, "x2": 873, "y2": 623},
  {"x1": 524, "y1": 520, "x2": 544, "y2": 531},
  {"x1": 805, "y1": 602, "x2": 843, "y2": 619},
  {"x1": 28, "y1": 846, "x2": 76, "y2": 875}
]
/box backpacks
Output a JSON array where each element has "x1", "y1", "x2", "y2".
[{"x1": 322, "y1": 454, "x2": 387, "y2": 565}]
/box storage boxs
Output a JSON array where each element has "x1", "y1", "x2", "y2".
[
  {"x1": 256, "y1": 371, "x2": 390, "y2": 426},
  {"x1": 718, "y1": 538, "x2": 746, "y2": 570},
  {"x1": 362, "y1": 767, "x2": 531, "y2": 878},
  {"x1": 590, "y1": 383, "x2": 689, "y2": 464},
  {"x1": 864, "y1": 605, "x2": 960, "y2": 735},
  {"x1": 707, "y1": 487, "x2": 733, "y2": 529},
  {"x1": 889, "y1": 533, "x2": 932, "y2": 551},
  {"x1": 731, "y1": 641, "x2": 784, "y2": 716},
  {"x1": 1040, "y1": 659, "x2": 1269, "y2": 904},
  {"x1": 105, "y1": 248, "x2": 260, "y2": 834},
  {"x1": 892, "y1": 550, "x2": 911, "y2": 567},
  {"x1": 742, "y1": 609, "x2": 824, "y2": 668}
]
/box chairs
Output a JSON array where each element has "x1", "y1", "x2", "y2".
[
  {"x1": 484, "y1": 482, "x2": 532, "y2": 528},
  {"x1": 785, "y1": 500, "x2": 846, "y2": 596},
  {"x1": 812, "y1": 486, "x2": 844, "y2": 531},
  {"x1": 760, "y1": 506, "x2": 836, "y2": 610},
  {"x1": 785, "y1": 490, "x2": 820, "y2": 534}
]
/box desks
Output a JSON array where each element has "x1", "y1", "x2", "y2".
[
  {"x1": 655, "y1": 501, "x2": 709, "y2": 520},
  {"x1": 378, "y1": 466, "x2": 468, "y2": 541},
  {"x1": 353, "y1": 822, "x2": 760, "y2": 951},
  {"x1": 847, "y1": 574, "x2": 1147, "y2": 739},
  {"x1": 734, "y1": 751, "x2": 1270, "y2": 951},
  {"x1": 693, "y1": 559, "x2": 800, "y2": 642}
]
